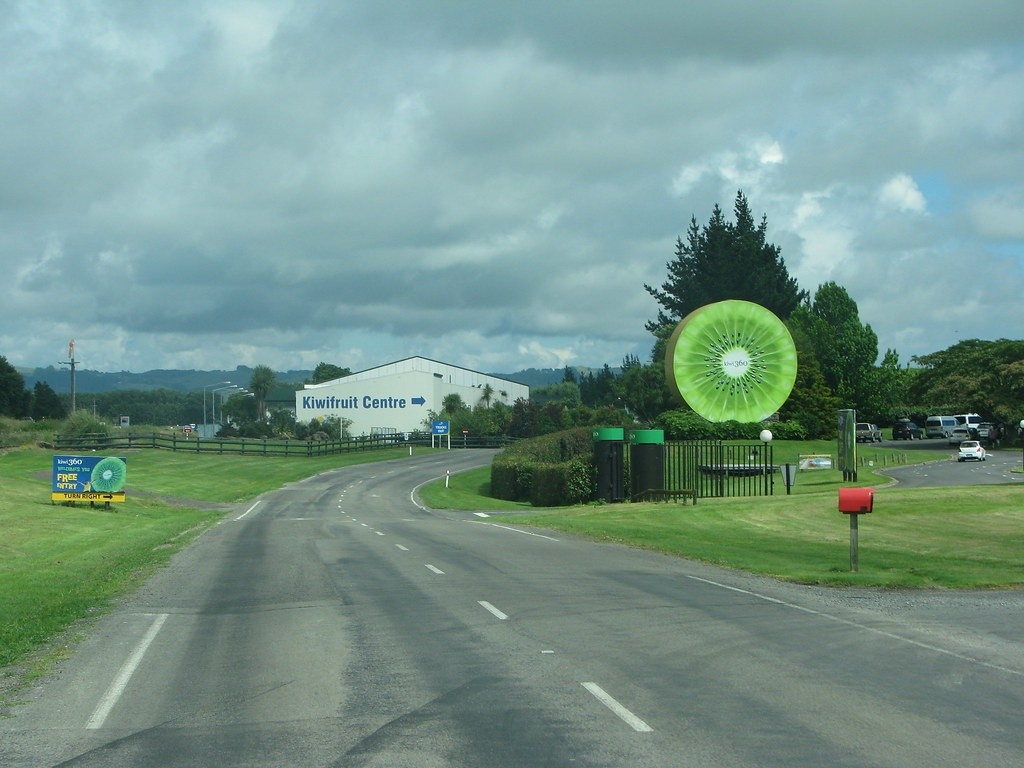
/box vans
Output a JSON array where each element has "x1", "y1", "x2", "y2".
[
  {"x1": 925, "y1": 416, "x2": 962, "y2": 438},
  {"x1": 953, "y1": 414, "x2": 983, "y2": 431}
]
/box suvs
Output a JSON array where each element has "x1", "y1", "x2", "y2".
[
  {"x1": 892, "y1": 422, "x2": 924, "y2": 440},
  {"x1": 977, "y1": 421, "x2": 999, "y2": 439},
  {"x1": 856, "y1": 422, "x2": 883, "y2": 443}
]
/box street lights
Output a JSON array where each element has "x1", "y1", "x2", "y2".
[{"x1": 203, "y1": 380, "x2": 255, "y2": 438}]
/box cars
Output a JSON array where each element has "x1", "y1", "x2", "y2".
[
  {"x1": 185, "y1": 426, "x2": 191, "y2": 432},
  {"x1": 190, "y1": 424, "x2": 196, "y2": 428},
  {"x1": 958, "y1": 440, "x2": 986, "y2": 462}
]
